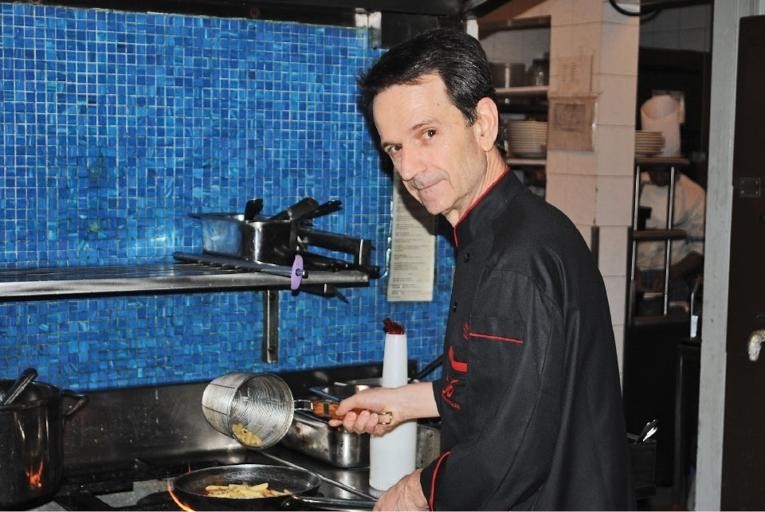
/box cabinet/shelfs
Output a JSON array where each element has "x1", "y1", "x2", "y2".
[{"x1": 629, "y1": 157, "x2": 692, "y2": 329}]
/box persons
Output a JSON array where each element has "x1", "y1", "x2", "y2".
[
  {"x1": 624, "y1": 164, "x2": 706, "y2": 312},
  {"x1": 329, "y1": 28, "x2": 639, "y2": 511}
]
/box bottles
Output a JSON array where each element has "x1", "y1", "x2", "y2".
[
  {"x1": 370, "y1": 327, "x2": 420, "y2": 491},
  {"x1": 527, "y1": 58, "x2": 550, "y2": 87}
]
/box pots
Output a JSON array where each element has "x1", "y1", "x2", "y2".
[
  {"x1": 0, "y1": 378, "x2": 89, "y2": 511},
  {"x1": 172, "y1": 463, "x2": 377, "y2": 511}
]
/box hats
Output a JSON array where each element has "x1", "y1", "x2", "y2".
[{"x1": 640, "y1": 95, "x2": 680, "y2": 157}]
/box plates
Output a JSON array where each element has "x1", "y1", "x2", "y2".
[
  {"x1": 634, "y1": 129, "x2": 666, "y2": 157},
  {"x1": 505, "y1": 119, "x2": 549, "y2": 160}
]
